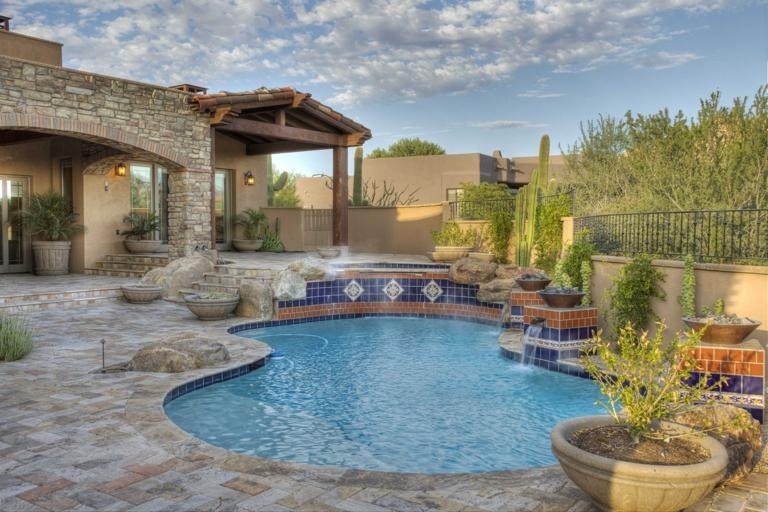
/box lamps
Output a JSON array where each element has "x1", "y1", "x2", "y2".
[
  {"x1": 244, "y1": 170, "x2": 255, "y2": 186},
  {"x1": 113, "y1": 164, "x2": 128, "y2": 176}
]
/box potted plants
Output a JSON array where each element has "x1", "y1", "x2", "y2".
[
  {"x1": 116, "y1": 210, "x2": 164, "y2": 253},
  {"x1": 19, "y1": 192, "x2": 85, "y2": 274},
  {"x1": 426, "y1": 221, "x2": 480, "y2": 257},
  {"x1": 550, "y1": 319, "x2": 730, "y2": 512},
  {"x1": 231, "y1": 209, "x2": 267, "y2": 252}
]
